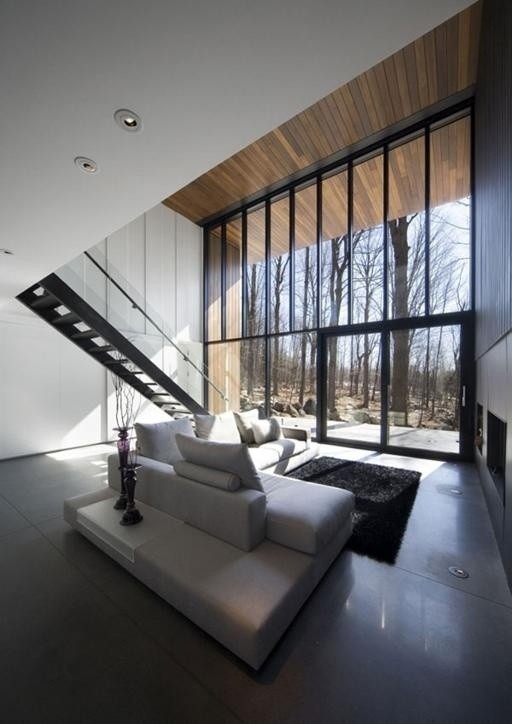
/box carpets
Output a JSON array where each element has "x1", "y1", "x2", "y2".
[{"x1": 280, "y1": 456, "x2": 424, "y2": 566}]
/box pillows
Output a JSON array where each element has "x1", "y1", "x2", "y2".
[
  {"x1": 234, "y1": 405, "x2": 267, "y2": 445},
  {"x1": 250, "y1": 416, "x2": 285, "y2": 444},
  {"x1": 132, "y1": 415, "x2": 195, "y2": 463},
  {"x1": 193, "y1": 408, "x2": 243, "y2": 444},
  {"x1": 174, "y1": 432, "x2": 266, "y2": 494}
]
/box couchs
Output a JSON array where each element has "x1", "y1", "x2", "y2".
[
  {"x1": 60, "y1": 450, "x2": 362, "y2": 674},
  {"x1": 128, "y1": 402, "x2": 322, "y2": 477}
]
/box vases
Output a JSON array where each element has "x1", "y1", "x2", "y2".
[
  {"x1": 117, "y1": 464, "x2": 148, "y2": 528},
  {"x1": 114, "y1": 425, "x2": 134, "y2": 510}
]
